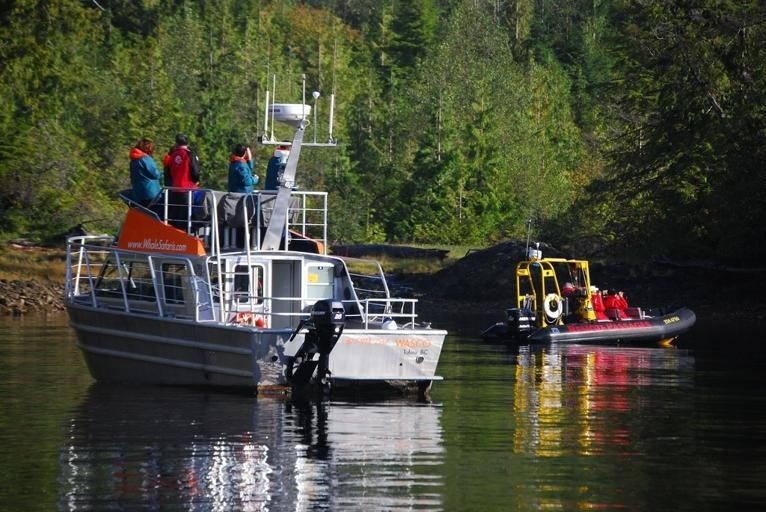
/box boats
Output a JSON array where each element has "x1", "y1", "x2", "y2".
[
  {"x1": 64, "y1": 74, "x2": 448, "y2": 404},
  {"x1": 479, "y1": 219, "x2": 697, "y2": 343}
]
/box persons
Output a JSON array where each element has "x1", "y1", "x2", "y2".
[
  {"x1": 562, "y1": 282, "x2": 628, "y2": 319},
  {"x1": 264, "y1": 140, "x2": 292, "y2": 191},
  {"x1": 162, "y1": 133, "x2": 200, "y2": 193},
  {"x1": 227, "y1": 143, "x2": 260, "y2": 192},
  {"x1": 129, "y1": 139, "x2": 161, "y2": 205}
]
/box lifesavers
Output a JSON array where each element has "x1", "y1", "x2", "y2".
[
  {"x1": 230, "y1": 311, "x2": 267, "y2": 329},
  {"x1": 544, "y1": 293, "x2": 563, "y2": 318}
]
